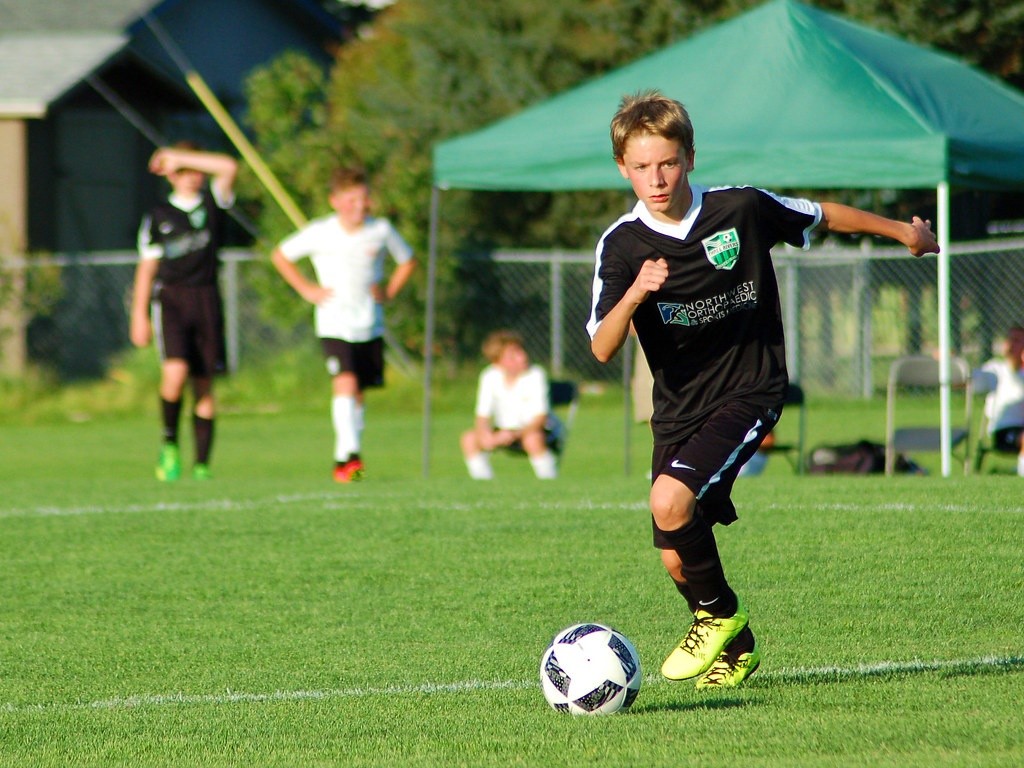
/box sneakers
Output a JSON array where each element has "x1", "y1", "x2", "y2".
[
  {"x1": 695, "y1": 641, "x2": 760, "y2": 688},
  {"x1": 662, "y1": 610, "x2": 749, "y2": 679}
]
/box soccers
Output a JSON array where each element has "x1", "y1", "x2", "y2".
[{"x1": 537, "y1": 620, "x2": 645, "y2": 720}]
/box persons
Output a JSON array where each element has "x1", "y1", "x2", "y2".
[
  {"x1": 458, "y1": 330, "x2": 558, "y2": 480},
  {"x1": 584, "y1": 89, "x2": 941, "y2": 691},
  {"x1": 269, "y1": 166, "x2": 418, "y2": 483},
  {"x1": 980, "y1": 324, "x2": 1024, "y2": 477},
  {"x1": 128, "y1": 137, "x2": 238, "y2": 482}
]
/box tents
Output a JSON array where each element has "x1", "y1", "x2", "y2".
[{"x1": 420, "y1": 0, "x2": 1024, "y2": 478}]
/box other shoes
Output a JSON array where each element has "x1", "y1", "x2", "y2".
[
  {"x1": 334, "y1": 461, "x2": 366, "y2": 484},
  {"x1": 193, "y1": 463, "x2": 210, "y2": 479},
  {"x1": 155, "y1": 444, "x2": 181, "y2": 481}
]
